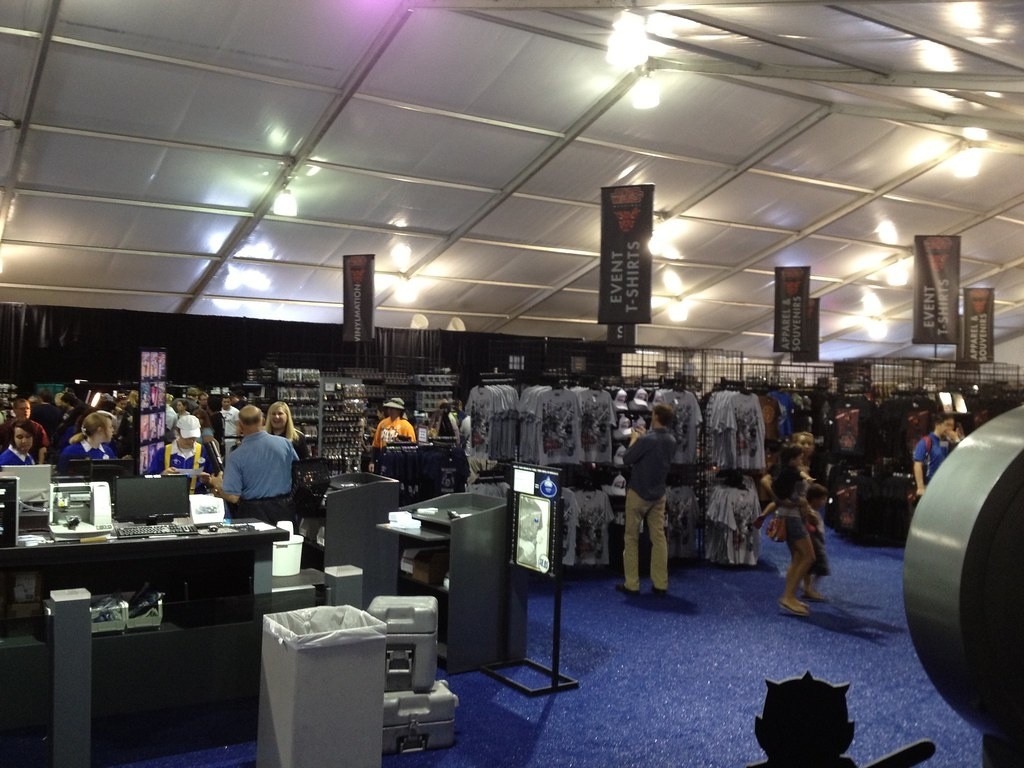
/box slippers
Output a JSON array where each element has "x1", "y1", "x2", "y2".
[
  {"x1": 779, "y1": 599, "x2": 808, "y2": 616},
  {"x1": 801, "y1": 590, "x2": 826, "y2": 602}
]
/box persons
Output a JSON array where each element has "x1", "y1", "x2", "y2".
[
  {"x1": 0, "y1": 420, "x2": 38, "y2": 466},
  {"x1": 165, "y1": 387, "x2": 248, "y2": 468},
  {"x1": 752, "y1": 443, "x2": 816, "y2": 527},
  {"x1": 0, "y1": 386, "x2": 139, "y2": 464},
  {"x1": 799, "y1": 484, "x2": 832, "y2": 602},
  {"x1": 460, "y1": 415, "x2": 471, "y2": 442},
  {"x1": 208, "y1": 404, "x2": 300, "y2": 534},
  {"x1": 0, "y1": 395, "x2": 49, "y2": 464},
  {"x1": 265, "y1": 401, "x2": 311, "y2": 459},
  {"x1": 616, "y1": 404, "x2": 677, "y2": 594},
  {"x1": 440, "y1": 399, "x2": 461, "y2": 446},
  {"x1": 912, "y1": 414, "x2": 966, "y2": 508},
  {"x1": 57, "y1": 411, "x2": 116, "y2": 471},
  {"x1": 761, "y1": 431, "x2": 830, "y2": 616},
  {"x1": 146, "y1": 413, "x2": 213, "y2": 495},
  {"x1": 369, "y1": 394, "x2": 416, "y2": 473}
]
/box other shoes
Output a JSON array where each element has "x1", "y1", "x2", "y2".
[
  {"x1": 616, "y1": 583, "x2": 635, "y2": 594},
  {"x1": 652, "y1": 586, "x2": 667, "y2": 597},
  {"x1": 753, "y1": 515, "x2": 766, "y2": 528},
  {"x1": 806, "y1": 524, "x2": 816, "y2": 532}
]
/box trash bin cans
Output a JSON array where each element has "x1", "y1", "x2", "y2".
[{"x1": 256, "y1": 605, "x2": 387, "y2": 768}]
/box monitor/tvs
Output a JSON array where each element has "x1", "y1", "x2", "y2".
[{"x1": 1, "y1": 459, "x2": 191, "y2": 526}]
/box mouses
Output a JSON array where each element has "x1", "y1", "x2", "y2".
[{"x1": 209, "y1": 524, "x2": 218, "y2": 532}]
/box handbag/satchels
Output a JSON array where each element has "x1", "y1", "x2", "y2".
[{"x1": 767, "y1": 517, "x2": 786, "y2": 542}]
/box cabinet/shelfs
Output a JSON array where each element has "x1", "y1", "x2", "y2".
[
  {"x1": 240, "y1": 368, "x2": 459, "y2": 478},
  {"x1": 297, "y1": 472, "x2": 528, "y2": 674}
]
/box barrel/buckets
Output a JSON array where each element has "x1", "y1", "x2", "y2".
[{"x1": 273, "y1": 535, "x2": 304, "y2": 577}]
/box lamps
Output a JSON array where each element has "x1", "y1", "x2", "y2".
[
  {"x1": 606, "y1": 9, "x2": 660, "y2": 109},
  {"x1": 447, "y1": 317, "x2": 466, "y2": 331},
  {"x1": 411, "y1": 314, "x2": 429, "y2": 329},
  {"x1": 274, "y1": 190, "x2": 298, "y2": 217}
]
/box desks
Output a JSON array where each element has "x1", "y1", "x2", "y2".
[{"x1": 0, "y1": 520, "x2": 329, "y2": 729}]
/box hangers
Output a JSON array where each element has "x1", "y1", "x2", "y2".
[{"x1": 387, "y1": 373, "x2": 1024, "y2": 495}]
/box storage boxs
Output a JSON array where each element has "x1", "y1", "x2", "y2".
[
  {"x1": 367, "y1": 596, "x2": 438, "y2": 693},
  {"x1": 89, "y1": 591, "x2": 163, "y2": 633},
  {"x1": 381, "y1": 680, "x2": 454, "y2": 755}
]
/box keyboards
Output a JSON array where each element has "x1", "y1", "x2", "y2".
[{"x1": 116, "y1": 525, "x2": 200, "y2": 540}]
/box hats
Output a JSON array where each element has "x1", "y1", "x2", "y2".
[
  {"x1": 383, "y1": 397, "x2": 405, "y2": 410},
  {"x1": 176, "y1": 415, "x2": 202, "y2": 438}
]
